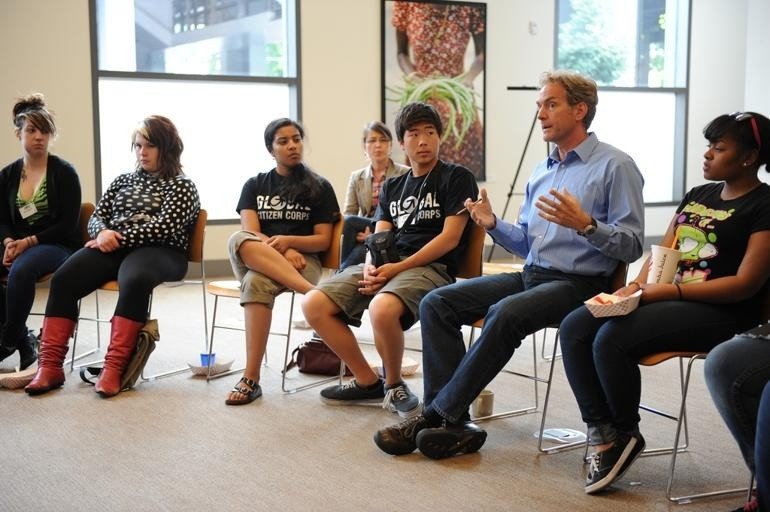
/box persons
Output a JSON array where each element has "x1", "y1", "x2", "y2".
[
  {"x1": 342, "y1": 121, "x2": 413, "y2": 219},
  {"x1": 561, "y1": 110, "x2": 770, "y2": 494},
  {"x1": 225, "y1": 116, "x2": 342, "y2": 405},
  {"x1": 373, "y1": 69, "x2": 645, "y2": 459},
  {"x1": 300, "y1": 102, "x2": 479, "y2": 420},
  {"x1": 25, "y1": 114, "x2": 201, "y2": 398},
  {"x1": 0, "y1": 93, "x2": 82, "y2": 388},
  {"x1": 704, "y1": 317, "x2": 769, "y2": 512}
]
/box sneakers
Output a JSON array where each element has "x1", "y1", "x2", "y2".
[
  {"x1": 610, "y1": 431, "x2": 647, "y2": 486},
  {"x1": 18, "y1": 330, "x2": 40, "y2": 371},
  {"x1": 319, "y1": 375, "x2": 386, "y2": 406},
  {"x1": 728, "y1": 495, "x2": 762, "y2": 512},
  {"x1": 583, "y1": 431, "x2": 638, "y2": 494},
  {"x1": 415, "y1": 420, "x2": 489, "y2": 461},
  {"x1": 373, "y1": 411, "x2": 435, "y2": 456},
  {"x1": 0, "y1": 344, "x2": 16, "y2": 364},
  {"x1": 383, "y1": 378, "x2": 423, "y2": 419}
]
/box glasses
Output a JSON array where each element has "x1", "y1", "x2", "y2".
[{"x1": 729, "y1": 110, "x2": 762, "y2": 153}]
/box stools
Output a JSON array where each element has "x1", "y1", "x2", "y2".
[
  {"x1": 635, "y1": 349, "x2": 756, "y2": 504},
  {"x1": 461, "y1": 256, "x2": 628, "y2": 458}
]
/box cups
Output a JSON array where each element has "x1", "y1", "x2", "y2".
[
  {"x1": 647, "y1": 244, "x2": 684, "y2": 285},
  {"x1": 201, "y1": 351, "x2": 216, "y2": 366},
  {"x1": 472, "y1": 390, "x2": 494, "y2": 418}
]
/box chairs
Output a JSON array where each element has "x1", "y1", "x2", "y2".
[
  {"x1": 2, "y1": 202, "x2": 103, "y2": 369},
  {"x1": 206, "y1": 212, "x2": 346, "y2": 395},
  {"x1": 68, "y1": 208, "x2": 211, "y2": 381},
  {"x1": 337, "y1": 215, "x2": 485, "y2": 409}
]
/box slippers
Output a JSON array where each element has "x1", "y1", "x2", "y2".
[{"x1": 225, "y1": 375, "x2": 263, "y2": 406}]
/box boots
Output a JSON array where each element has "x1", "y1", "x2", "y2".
[
  {"x1": 94, "y1": 314, "x2": 145, "y2": 398},
  {"x1": 24, "y1": 316, "x2": 77, "y2": 394}
]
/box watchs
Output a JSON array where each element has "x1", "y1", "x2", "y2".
[{"x1": 576, "y1": 218, "x2": 597, "y2": 237}]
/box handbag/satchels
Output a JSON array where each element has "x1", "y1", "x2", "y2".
[
  {"x1": 363, "y1": 229, "x2": 401, "y2": 269},
  {"x1": 118, "y1": 318, "x2": 160, "y2": 394},
  {"x1": 280, "y1": 336, "x2": 356, "y2": 377}
]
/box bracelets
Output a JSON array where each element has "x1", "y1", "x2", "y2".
[
  {"x1": 629, "y1": 280, "x2": 641, "y2": 288},
  {"x1": 674, "y1": 282, "x2": 682, "y2": 301}
]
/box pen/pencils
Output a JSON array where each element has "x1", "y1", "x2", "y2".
[{"x1": 456, "y1": 198, "x2": 483, "y2": 216}]
